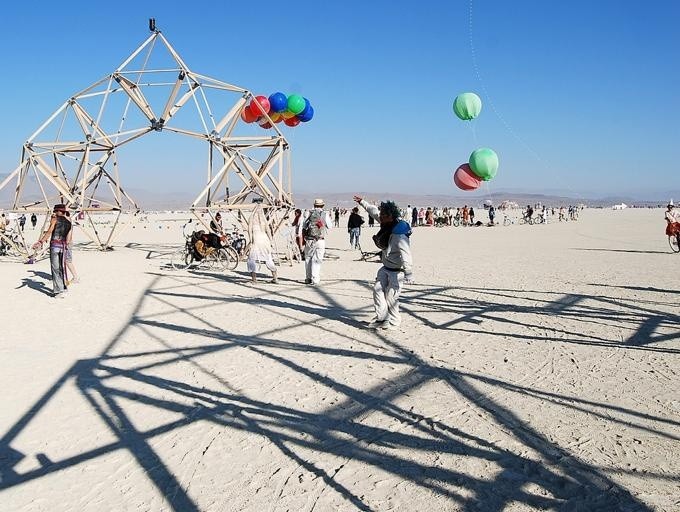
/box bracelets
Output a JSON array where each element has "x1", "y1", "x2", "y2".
[{"x1": 37, "y1": 239, "x2": 44, "y2": 245}]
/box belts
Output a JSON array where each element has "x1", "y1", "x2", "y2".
[{"x1": 384, "y1": 265, "x2": 405, "y2": 272}]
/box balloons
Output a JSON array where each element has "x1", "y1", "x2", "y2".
[
  {"x1": 240, "y1": 92, "x2": 314, "y2": 130},
  {"x1": 451, "y1": 93, "x2": 483, "y2": 120},
  {"x1": 453, "y1": 162, "x2": 482, "y2": 192},
  {"x1": 469, "y1": 146, "x2": 500, "y2": 181}
]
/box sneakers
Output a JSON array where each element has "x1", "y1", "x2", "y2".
[
  {"x1": 305, "y1": 278, "x2": 321, "y2": 288},
  {"x1": 369, "y1": 320, "x2": 396, "y2": 329}
]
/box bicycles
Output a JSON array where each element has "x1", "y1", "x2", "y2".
[
  {"x1": 171, "y1": 218, "x2": 246, "y2": 272},
  {"x1": 0, "y1": 227, "x2": 27, "y2": 258},
  {"x1": 453, "y1": 213, "x2": 544, "y2": 228},
  {"x1": 669, "y1": 229, "x2": 680, "y2": 253}
]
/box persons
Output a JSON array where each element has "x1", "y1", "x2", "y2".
[
  {"x1": 32, "y1": 204, "x2": 71, "y2": 297},
  {"x1": 304, "y1": 198, "x2": 333, "y2": 286},
  {"x1": 524, "y1": 204, "x2": 534, "y2": 220},
  {"x1": 30, "y1": 212, "x2": 37, "y2": 230},
  {"x1": 291, "y1": 209, "x2": 306, "y2": 254},
  {"x1": 353, "y1": 193, "x2": 416, "y2": 330},
  {"x1": 665, "y1": 205, "x2": 680, "y2": 245},
  {"x1": 208, "y1": 211, "x2": 224, "y2": 237},
  {"x1": 303, "y1": 209, "x2": 307, "y2": 218},
  {"x1": 348, "y1": 206, "x2": 365, "y2": 249},
  {"x1": 329, "y1": 204, "x2": 475, "y2": 227},
  {"x1": 65, "y1": 211, "x2": 82, "y2": 283},
  {"x1": 17, "y1": 212, "x2": 26, "y2": 231},
  {"x1": 540, "y1": 204, "x2": 578, "y2": 222},
  {"x1": 247, "y1": 204, "x2": 281, "y2": 284},
  {"x1": 489, "y1": 205, "x2": 495, "y2": 223}
]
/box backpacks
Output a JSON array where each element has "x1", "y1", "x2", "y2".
[{"x1": 307, "y1": 210, "x2": 323, "y2": 238}]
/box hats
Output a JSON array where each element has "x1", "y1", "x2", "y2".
[
  {"x1": 667, "y1": 200, "x2": 675, "y2": 207},
  {"x1": 53, "y1": 204, "x2": 66, "y2": 213},
  {"x1": 314, "y1": 198, "x2": 325, "y2": 206}
]
